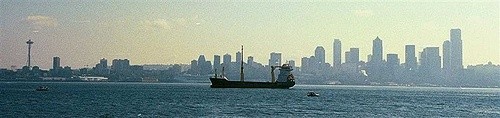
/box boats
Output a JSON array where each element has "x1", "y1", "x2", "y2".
[{"x1": 209, "y1": 45, "x2": 295, "y2": 88}]
